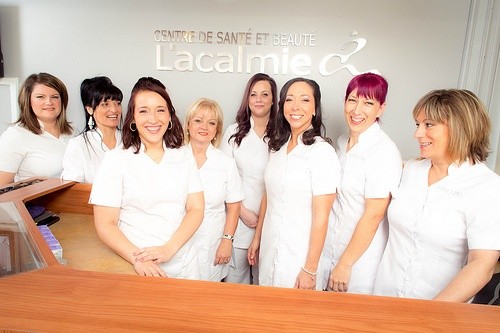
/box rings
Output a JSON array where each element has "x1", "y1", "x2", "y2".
[
  {"x1": 223, "y1": 261, "x2": 228, "y2": 263},
  {"x1": 338, "y1": 285, "x2": 344, "y2": 288}
]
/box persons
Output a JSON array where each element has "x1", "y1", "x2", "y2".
[
  {"x1": 0, "y1": 73, "x2": 85, "y2": 189},
  {"x1": 87, "y1": 72, "x2": 500, "y2": 307},
  {"x1": 61, "y1": 76, "x2": 123, "y2": 184}
]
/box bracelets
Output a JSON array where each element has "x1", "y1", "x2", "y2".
[{"x1": 300, "y1": 266, "x2": 319, "y2": 280}]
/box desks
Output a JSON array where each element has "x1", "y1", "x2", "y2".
[{"x1": 0, "y1": 181, "x2": 500, "y2": 333}]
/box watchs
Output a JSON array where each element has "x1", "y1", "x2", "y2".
[{"x1": 221, "y1": 234, "x2": 234, "y2": 243}]
